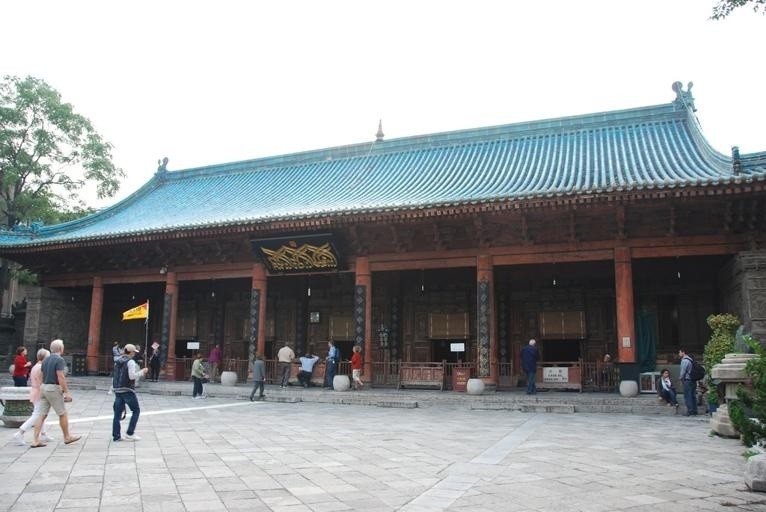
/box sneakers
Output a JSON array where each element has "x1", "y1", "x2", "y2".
[
  {"x1": 16, "y1": 432, "x2": 53, "y2": 447},
  {"x1": 64, "y1": 435, "x2": 81, "y2": 443},
  {"x1": 125, "y1": 433, "x2": 141, "y2": 440}
]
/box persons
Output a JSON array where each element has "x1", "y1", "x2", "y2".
[
  {"x1": 149, "y1": 349, "x2": 161, "y2": 382},
  {"x1": 108, "y1": 342, "x2": 119, "y2": 394},
  {"x1": 14, "y1": 348, "x2": 54, "y2": 445},
  {"x1": 191, "y1": 354, "x2": 206, "y2": 399},
  {"x1": 678, "y1": 346, "x2": 697, "y2": 415},
  {"x1": 520, "y1": 338, "x2": 540, "y2": 394},
  {"x1": 297, "y1": 352, "x2": 320, "y2": 388},
  {"x1": 655, "y1": 370, "x2": 678, "y2": 407},
  {"x1": 249, "y1": 355, "x2": 266, "y2": 401},
  {"x1": 134, "y1": 344, "x2": 143, "y2": 388},
  {"x1": 277, "y1": 342, "x2": 295, "y2": 389},
  {"x1": 31, "y1": 339, "x2": 81, "y2": 447},
  {"x1": 323, "y1": 339, "x2": 336, "y2": 389},
  {"x1": 13, "y1": 346, "x2": 32, "y2": 386},
  {"x1": 349, "y1": 346, "x2": 364, "y2": 390},
  {"x1": 208, "y1": 344, "x2": 221, "y2": 383},
  {"x1": 112, "y1": 343, "x2": 148, "y2": 442}
]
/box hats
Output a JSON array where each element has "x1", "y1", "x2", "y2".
[{"x1": 125, "y1": 344, "x2": 139, "y2": 353}]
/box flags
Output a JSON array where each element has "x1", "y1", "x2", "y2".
[{"x1": 122, "y1": 303, "x2": 147, "y2": 320}]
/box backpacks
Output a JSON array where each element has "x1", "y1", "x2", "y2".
[{"x1": 684, "y1": 354, "x2": 705, "y2": 380}]
